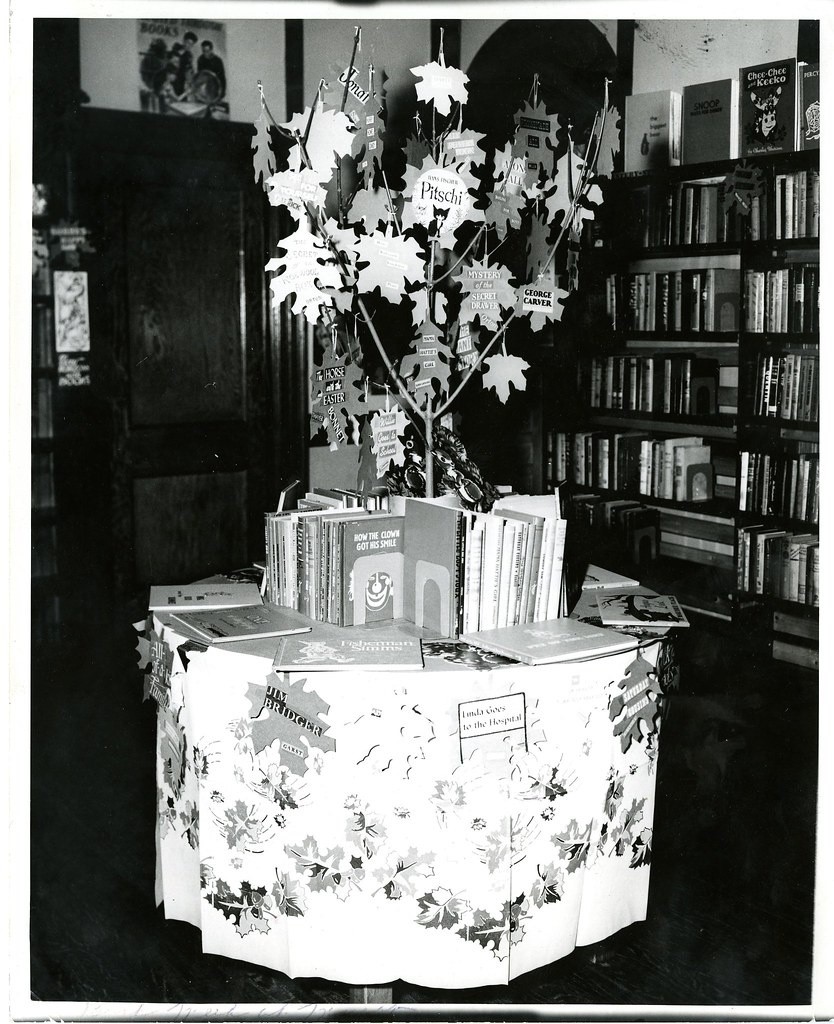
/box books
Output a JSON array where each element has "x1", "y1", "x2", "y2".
[
  {"x1": 169, "y1": 604, "x2": 313, "y2": 643},
  {"x1": 546, "y1": 166, "x2": 819, "y2": 673},
  {"x1": 32, "y1": 227, "x2": 60, "y2": 647},
  {"x1": 260, "y1": 480, "x2": 567, "y2": 644},
  {"x1": 460, "y1": 616, "x2": 641, "y2": 666},
  {"x1": 624, "y1": 91, "x2": 683, "y2": 172},
  {"x1": 148, "y1": 583, "x2": 264, "y2": 611},
  {"x1": 799, "y1": 63, "x2": 819, "y2": 151},
  {"x1": 272, "y1": 638, "x2": 423, "y2": 672},
  {"x1": 738, "y1": 58, "x2": 800, "y2": 158},
  {"x1": 681, "y1": 79, "x2": 739, "y2": 165}
]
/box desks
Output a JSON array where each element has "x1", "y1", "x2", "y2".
[{"x1": 151, "y1": 568, "x2": 674, "y2": 991}]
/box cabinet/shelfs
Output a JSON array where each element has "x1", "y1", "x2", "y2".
[{"x1": 455, "y1": 148, "x2": 818, "y2": 671}]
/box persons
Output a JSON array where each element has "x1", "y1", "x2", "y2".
[
  {"x1": 57, "y1": 275, "x2": 85, "y2": 348},
  {"x1": 140, "y1": 30, "x2": 226, "y2": 102}
]
